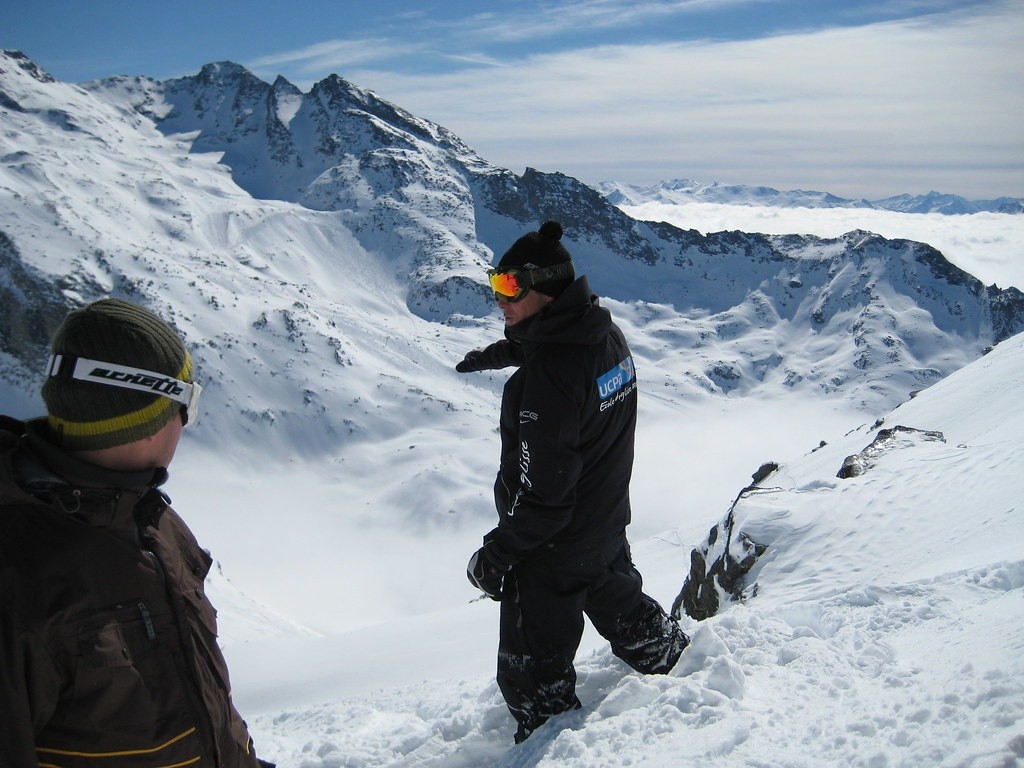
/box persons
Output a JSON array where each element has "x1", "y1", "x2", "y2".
[
  {"x1": 454, "y1": 221, "x2": 692, "y2": 749},
  {"x1": 0, "y1": 299, "x2": 277, "y2": 768}
]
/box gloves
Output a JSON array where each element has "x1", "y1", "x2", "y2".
[
  {"x1": 455, "y1": 360, "x2": 469, "y2": 372},
  {"x1": 466, "y1": 544, "x2": 505, "y2": 601}
]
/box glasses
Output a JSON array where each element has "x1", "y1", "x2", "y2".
[
  {"x1": 492, "y1": 268, "x2": 534, "y2": 303},
  {"x1": 180, "y1": 379, "x2": 202, "y2": 429}
]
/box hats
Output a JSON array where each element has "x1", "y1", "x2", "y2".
[
  {"x1": 37, "y1": 294, "x2": 197, "y2": 448},
  {"x1": 499, "y1": 221, "x2": 575, "y2": 298}
]
